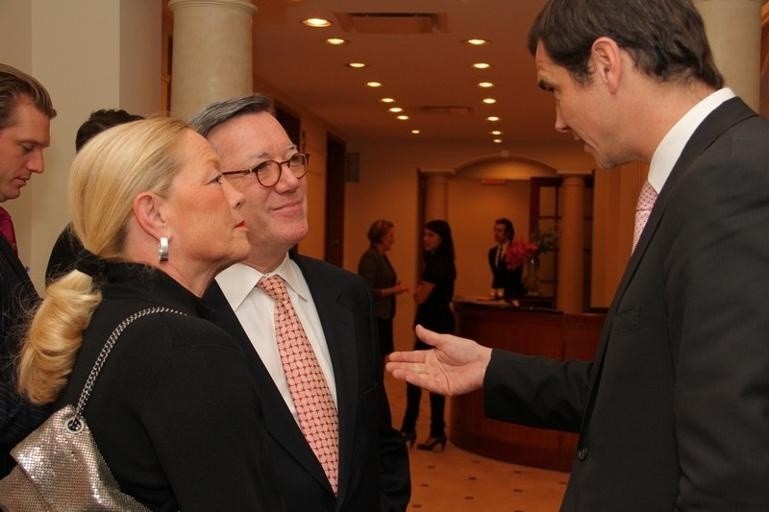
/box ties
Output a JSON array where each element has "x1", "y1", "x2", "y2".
[
  {"x1": 498, "y1": 248, "x2": 503, "y2": 263},
  {"x1": 632, "y1": 181, "x2": 659, "y2": 255},
  {"x1": 257, "y1": 274, "x2": 340, "y2": 497}
]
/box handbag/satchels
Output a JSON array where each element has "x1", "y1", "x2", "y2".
[{"x1": 0, "y1": 404, "x2": 154, "y2": 512}]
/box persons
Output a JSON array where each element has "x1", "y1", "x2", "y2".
[
  {"x1": 183, "y1": 93, "x2": 411, "y2": 512},
  {"x1": 354, "y1": 221, "x2": 409, "y2": 370},
  {"x1": 20, "y1": 114, "x2": 290, "y2": 512},
  {"x1": 1, "y1": 63, "x2": 57, "y2": 480},
  {"x1": 397, "y1": 219, "x2": 457, "y2": 451},
  {"x1": 44, "y1": 104, "x2": 147, "y2": 286},
  {"x1": 486, "y1": 218, "x2": 529, "y2": 299},
  {"x1": 383, "y1": 0, "x2": 769, "y2": 512}
]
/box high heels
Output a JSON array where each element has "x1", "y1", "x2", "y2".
[
  {"x1": 403, "y1": 430, "x2": 416, "y2": 447},
  {"x1": 417, "y1": 434, "x2": 446, "y2": 450}
]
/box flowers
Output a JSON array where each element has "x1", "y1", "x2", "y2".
[{"x1": 505, "y1": 223, "x2": 558, "y2": 270}]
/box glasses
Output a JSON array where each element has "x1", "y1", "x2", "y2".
[{"x1": 223, "y1": 148, "x2": 310, "y2": 189}]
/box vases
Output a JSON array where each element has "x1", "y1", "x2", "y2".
[{"x1": 524, "y1": 258, "x2": 542, "y2": 295}]
